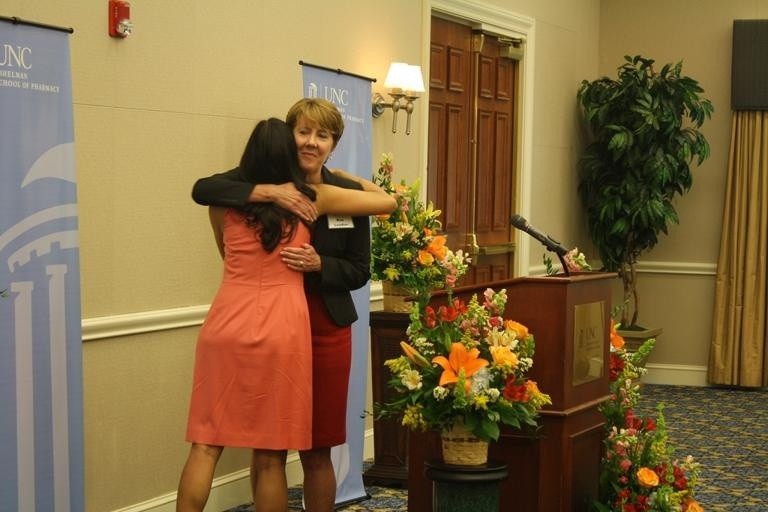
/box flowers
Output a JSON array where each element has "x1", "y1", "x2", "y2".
[
  {"x1": 358, "y1": 286, "x2": 554, "y2": 444},
  {"x1": 588, "y1": 298, "x2": 708, "y2": 512},
  {"x1": 370, "y1": 151, "x2": 471, "y2": 291}
]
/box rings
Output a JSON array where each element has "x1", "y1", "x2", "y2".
[{"x1": 299, "y1": 260, "x2": 305, "y2": 266}]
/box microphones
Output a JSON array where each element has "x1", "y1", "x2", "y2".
[{"x1": 510, "y1": 214, "x2": 568, "y2": 256}]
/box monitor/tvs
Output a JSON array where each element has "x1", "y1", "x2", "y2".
[{"x1": 730, "y1": 19, "x2": 768, "y2": 110}]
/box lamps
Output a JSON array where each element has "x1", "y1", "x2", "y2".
[{"x1": 368, "y1": 62, "x2": 424, "y2": 135}]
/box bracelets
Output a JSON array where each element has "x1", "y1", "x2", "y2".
[{"x1": 355, "y1": 175, "x2": 361, "y2": 182}]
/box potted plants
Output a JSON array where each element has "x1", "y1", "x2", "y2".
[{"x1": 575, "y1": 51, "x2": 716, "y2": 364}]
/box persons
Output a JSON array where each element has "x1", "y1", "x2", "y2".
[
  {"x1": 191, "y1": 97, "x2": 370, "y2": 511},
  {"x1": 173, "y1": 118, "x2": 399, "y2": 512}
]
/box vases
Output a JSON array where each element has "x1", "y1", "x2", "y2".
[
  {"x1": 440, "y1": 416, "x2": 490, "y2": 466},
  {"x1": 384, "y1": 280, "x2": 419, "y2": 314}
]
